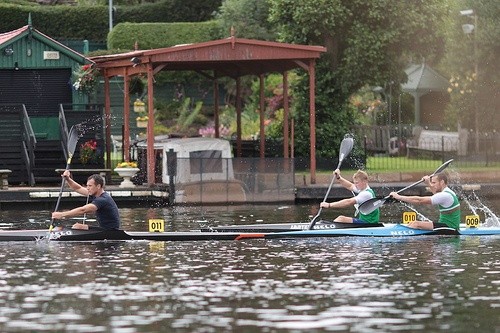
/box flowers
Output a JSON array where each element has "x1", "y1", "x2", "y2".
[
  {"x1": 79, "y1": 139, "x2": 100, "y2": 164},
  {"x1": 117, "y1": 161, "x2": 138, "y2": 168},
  {"x1": 71, "y1": 64, "x2": 99, "y2": 98},
  {"x1": 134, "y1": 102, "x2": 145, "y2": 106},
  {"x1": 136, "y1": 116, "x2": 149, "y2": 120}
]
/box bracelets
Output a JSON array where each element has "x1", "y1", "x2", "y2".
[{"x1": 337, "y1": 177, "x2": 340, "y2": 179}]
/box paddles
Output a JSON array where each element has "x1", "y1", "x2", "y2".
[
  {"x1": 358, "y1": 159, "x2": 454, "y2": 216},
  {"x1": 308, "y1": 138, "x2": 354, "y2": 230},
  {"x1": 48, "y1": 124, "x2": 78, "y2": 230}
]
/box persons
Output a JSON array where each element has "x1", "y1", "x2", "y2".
[
  {"x1": 52, "y1": 171, "x2": 120, "y2": 231},
  {"x1": 320, "y1": 169, "x2": 379, "y2": 223},
  {"x1": 389, "y1": 172, "x2": 460, "y2": 230}
]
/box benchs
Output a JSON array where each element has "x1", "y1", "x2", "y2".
[
  {"x1": 0, "y1": 170, "x2": 12, "y2": 190},
  {"x1": 55, "y1": 169, "x2": 111, "y2": 188}
]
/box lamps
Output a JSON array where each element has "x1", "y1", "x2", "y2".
[{"x1": 130, "y1": 57, "x2": 140, "y2": 67}]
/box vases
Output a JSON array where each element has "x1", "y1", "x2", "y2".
[
  {"x1": 133, "y1": 106, "x2": 145, "y2": 112},
  {"x1": 137, "y1": 121, "x2": 148, "y2": 128},
  {"x1": 114, "y1": 168, "x2": 140, "y2": 187}
]
